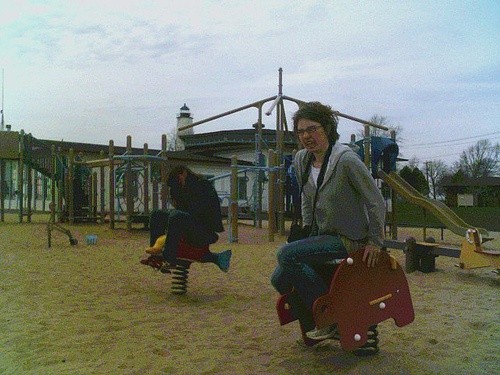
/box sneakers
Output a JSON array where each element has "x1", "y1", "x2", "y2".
[{"x1": 305, "y1": 325, "x2": 337, "y2": 341}]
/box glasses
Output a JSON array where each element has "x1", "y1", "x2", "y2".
[{"x1": 296, "y1": 126, "x2": 324, "y2": 135}]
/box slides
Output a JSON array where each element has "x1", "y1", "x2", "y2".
[{"x1": 377, "y1": 165, "x2": 491, "y2": 239}]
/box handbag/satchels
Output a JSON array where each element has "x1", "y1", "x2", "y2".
[{"x1": 287, "y1": 223, "x2": 319, "y2": 243}]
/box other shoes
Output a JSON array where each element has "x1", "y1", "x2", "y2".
[
  {"x1": 141, "y1": 255, "x2": 160, "y2": 265},
  {"x1": 149, "y1": 254, "x2": 176, "y2": 267}
]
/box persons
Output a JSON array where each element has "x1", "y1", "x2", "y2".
[
  {"x1": 140, "y1": 165, "x2": 224, "y2": 268},
  {"x1": 270, "y1": 102, "x2": 386, "y2": 340}
]
